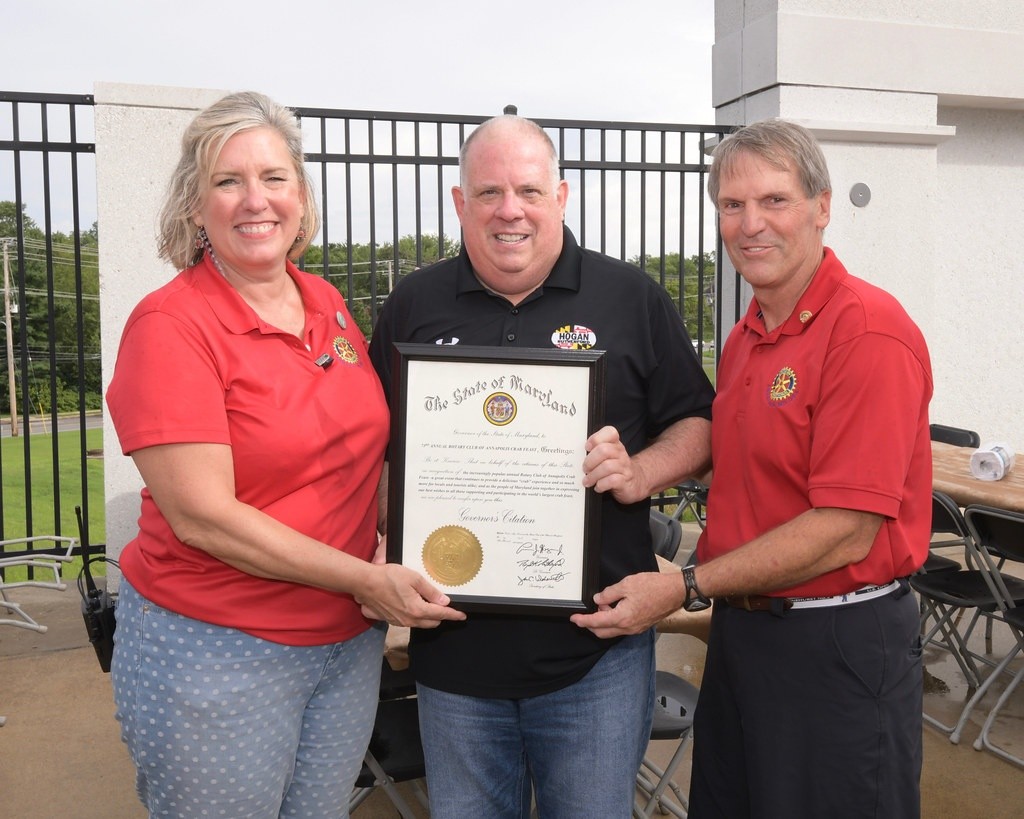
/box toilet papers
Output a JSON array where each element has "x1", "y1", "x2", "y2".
[{"x1": 969, "y1": 441, "x2": 1015, "y2": 482}]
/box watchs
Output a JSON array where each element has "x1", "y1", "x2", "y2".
[{"x1": 680, "y1": 564, "x2": 711, "y2": 612}]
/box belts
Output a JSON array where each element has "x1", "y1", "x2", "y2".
[{"x1": 724, "y1": 578, "x2": 901, "y2": 614}]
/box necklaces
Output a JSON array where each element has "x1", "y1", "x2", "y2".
[{"x1": 200, "y1": 228, "x2": 227, "y2": 279}]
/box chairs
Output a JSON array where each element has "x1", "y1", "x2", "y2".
[{"x1": 353, "y1": 424, "x2": 1024, "y2": 819}]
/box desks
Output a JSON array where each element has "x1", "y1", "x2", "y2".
[{"x1": 930, "y1": 440, "x2": 1024, "y2": 514}]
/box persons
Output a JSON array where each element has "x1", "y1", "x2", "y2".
[
  {"x1": 106, "y1": 90, "x2": 466, "y2": 819},
  {"x1": 569, "y1": 119, "x2": 934, "y2": 819},
  {"x1": 354, "y1": 115, "x2": 716, "y2": 819}
]
